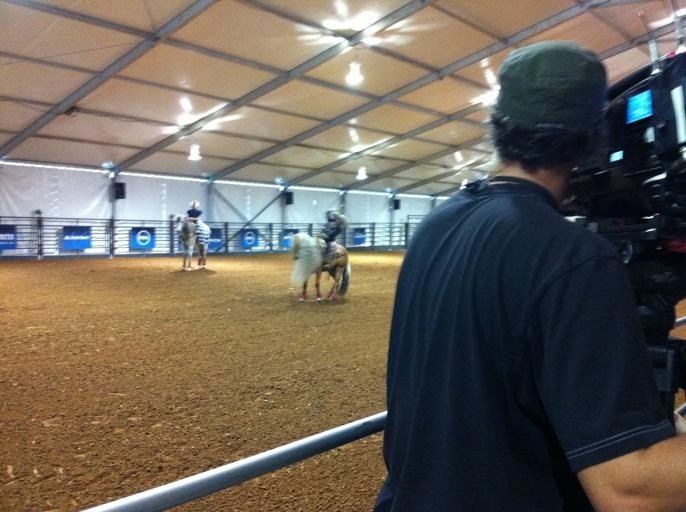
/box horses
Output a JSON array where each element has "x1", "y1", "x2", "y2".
[
  {"x1": 177, "y1": 219, "x2": 211, "y2": 271},
  {"x1": 290, "y1": 233, "x2": 352, "y2": 304}
]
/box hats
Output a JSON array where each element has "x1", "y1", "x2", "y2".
[{"x1": 494, "y1": 39, "x2": 606, "y2": 126}]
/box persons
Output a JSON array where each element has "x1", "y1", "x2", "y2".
[
  {"x1": 373, "y1": 41, "x2": 685, "y2": 512},
  {"x1": 185, "y1": 201, "x2": 204, "y2": 230},
  {"x1": 324, "y1": 209, "x2": 344, "y2": 257}
]
